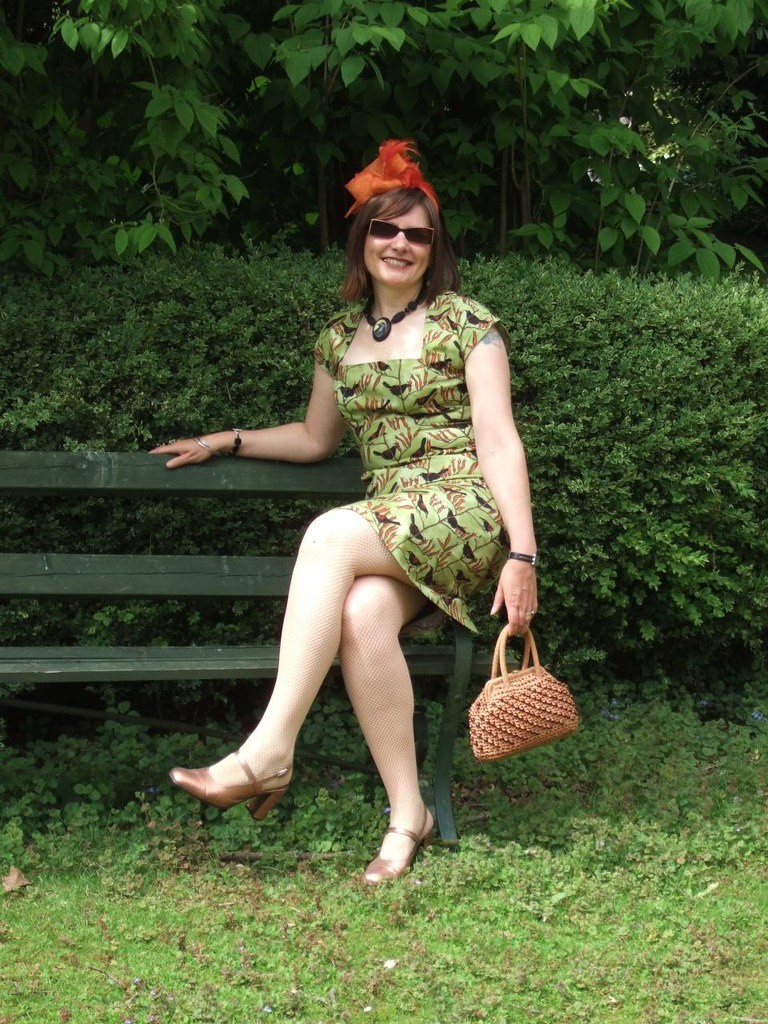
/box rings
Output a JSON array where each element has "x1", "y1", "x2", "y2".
[{"x1": 526, "y1": 610, "x2": 535, "y2": 618}]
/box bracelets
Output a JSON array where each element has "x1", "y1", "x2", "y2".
[{"x1": 194, "y1": 427, "x2": 244, "y2": 457}]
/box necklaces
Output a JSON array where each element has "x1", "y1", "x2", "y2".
[{"x1": 363, "y1": 283, "x2": 427, "y2": 341}]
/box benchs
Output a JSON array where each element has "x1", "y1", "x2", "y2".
[{"x1": 1, "y1": 448, "x2": 474, "y2": 846}]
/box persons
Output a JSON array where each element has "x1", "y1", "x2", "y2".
[{"x1": 149, "y1": 138, "x2": 539, "y2": 886}]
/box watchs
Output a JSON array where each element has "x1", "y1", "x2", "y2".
[{"x1": 506, "y1": 550, "x2": 540, "y2": 567}]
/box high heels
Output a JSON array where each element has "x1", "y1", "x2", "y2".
[
  {"x1": 169, "y1": 748, "x2": 293, "y2": 821},
  {"x1": 363, "y1": 805, "x2": 435, "y2": 888}
]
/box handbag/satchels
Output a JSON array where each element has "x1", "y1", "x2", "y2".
[{"x1": 470, "y1": 623, "x2": 579, "y2": 762}]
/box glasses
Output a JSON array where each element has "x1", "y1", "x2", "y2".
[{"x1": 367, "y1": 219, "x2": 434, "y2": 245}]
